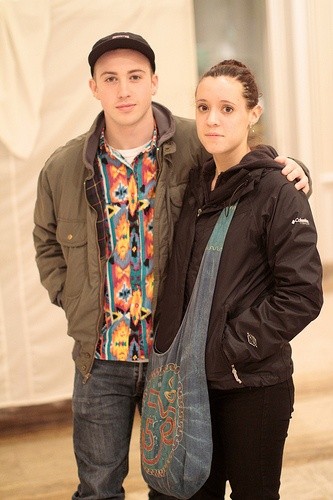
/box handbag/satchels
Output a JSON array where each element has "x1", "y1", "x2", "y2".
[{"x1": 138, "y1": 332, "x2": 214, "y2": 497}]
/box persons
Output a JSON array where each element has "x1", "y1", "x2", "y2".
[
  {"x1": 139, "y1": 57, "x2": 324, "y2": 500},
  {"x1": 31, "y1": 30, "x2": 312, "y2": 500}
]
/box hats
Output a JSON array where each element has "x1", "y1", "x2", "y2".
[{"x1": 88, "y1": 31, "x2": 156, "y2": 74}]
